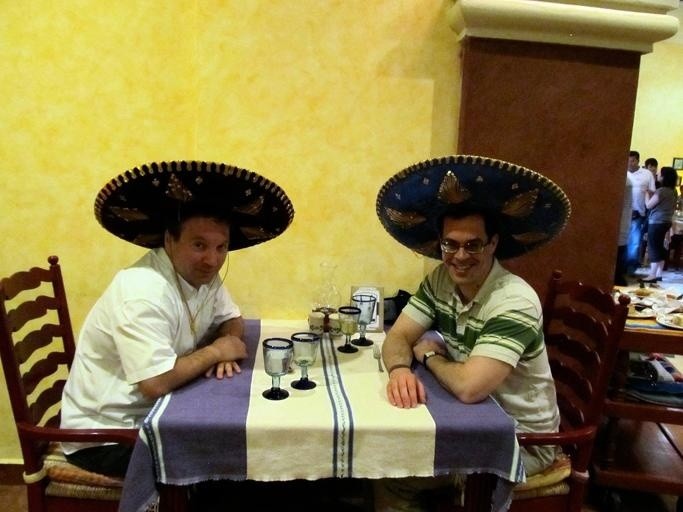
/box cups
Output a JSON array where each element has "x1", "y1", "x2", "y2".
[{"x1": 309, "y1": 311, "x2": 325, "y2": 339}]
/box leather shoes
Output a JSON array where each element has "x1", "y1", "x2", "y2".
[{"x1": 638, "y1": 276, "x2": 663, "y2": 282}]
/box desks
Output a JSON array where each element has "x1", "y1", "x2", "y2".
[{"x1": 146, "y1": 317, "x2": 517, "y2": 512}]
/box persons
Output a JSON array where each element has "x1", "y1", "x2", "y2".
[
  {"x1": 614, "y1": 149, "x2": 682, "y2": 287},
  {"x1": 368, "y1": 198, "x2": 563, "y2": 509},
  {"x1": 56, "y1": 198, "x2": 251, "y2": 511}
]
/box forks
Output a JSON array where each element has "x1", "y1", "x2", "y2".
[{"x1": 373, "y1": 346, "x2": 384, "y2": 373}]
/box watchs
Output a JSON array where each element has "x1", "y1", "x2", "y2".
[{"x1": 422, "y1": 349, "x2": 452, "y2": 369}]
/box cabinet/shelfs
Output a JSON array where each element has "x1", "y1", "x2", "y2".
[{"x1": 589, "y1": 286, "x2": 682, "y2": 497}]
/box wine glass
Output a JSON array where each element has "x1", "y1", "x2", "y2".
[
  {"x1": 338, "y1": 294, "x2": 376, "y2": 354},
  {"x1": 261, "y1": 331, "x2": 319, "y2": 401}
]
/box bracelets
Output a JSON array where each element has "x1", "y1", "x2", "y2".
[{"x1": 388, "y1": 363, "x2": 412, "y2": 380}]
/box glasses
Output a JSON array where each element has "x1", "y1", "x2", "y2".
[{"x1": 437, "y1": 238, "x2": 493, "y2": 254}]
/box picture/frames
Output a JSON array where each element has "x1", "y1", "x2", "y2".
[{"x1": 673, "y1": 157, "x2": 683, "y2": 170}]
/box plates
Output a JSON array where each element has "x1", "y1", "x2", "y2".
[{"x1": 615, "y1": 285, "x2": 683, "y2": 329}]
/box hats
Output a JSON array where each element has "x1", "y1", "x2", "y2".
[
  {"x1": 94, "y1": 160, "x2": 294, "y2": 250},
  {"x1": 376, "y1": 154, "x2": 572, "y2": 261}
]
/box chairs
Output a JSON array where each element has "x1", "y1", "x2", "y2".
[
  {"x1": 515, "y1": 268, "x2": 631, "y2": 512},
  {"x1": 0, "y1": 254, "x2": 141, "y2": 512}
]
